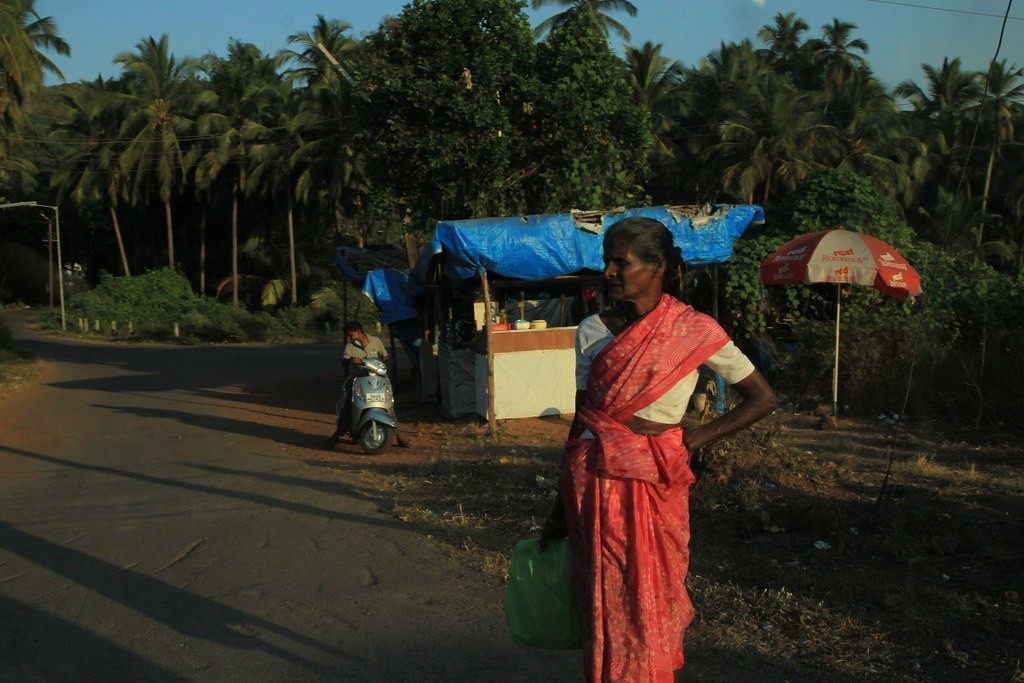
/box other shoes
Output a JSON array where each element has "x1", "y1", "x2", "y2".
[
  {"x1": 329, "y1": 432, "x2": 339, "y2": 446},
  {"x1": 397, "y1": 435, "x2": 410, "y2": 447}
]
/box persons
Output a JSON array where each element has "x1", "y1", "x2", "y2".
[
  {"x1": 325, "y1": 320, "x2": 414, "y2": 448},
  {"x1": 541, "y1": 217, "x2": 779, "y2": 683}
]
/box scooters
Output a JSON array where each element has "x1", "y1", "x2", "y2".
[{"x1": 331, "y1": 341, "x2": 399, "y2": 455}]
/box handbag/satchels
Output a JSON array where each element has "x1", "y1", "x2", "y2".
[{"x1": 503, "y1": 536, "x2": 583, "y2": 650}]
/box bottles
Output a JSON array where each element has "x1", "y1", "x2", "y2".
[
  {"x1": 496, "y1": 310, "x2": 500, "y2": 323},
  {"x1": 500, "y1": 309, "x2": 506, "y2": 324},
  {"x1": 491, "y1": 309, "x2": 496, "y2": 323}
]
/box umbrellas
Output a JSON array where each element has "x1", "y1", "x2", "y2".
[{"x1": 759, "y1": 225, "x2": 923, "y2": 420}]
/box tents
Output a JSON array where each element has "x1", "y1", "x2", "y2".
[{"x1": 335, "y1": 204, "x2": 766, "y2": 437}]
